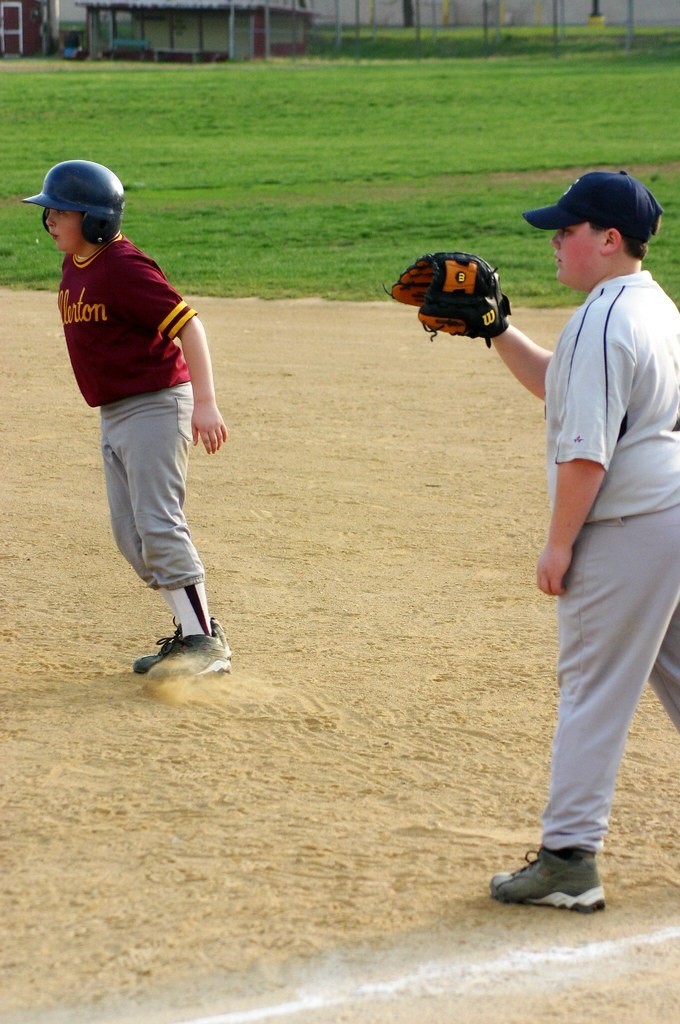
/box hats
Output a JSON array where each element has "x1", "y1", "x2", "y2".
[{"x1": 522, "y1": 170, "x2": 663, "y2": 243}]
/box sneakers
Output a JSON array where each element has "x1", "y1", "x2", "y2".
[
  {"x1": 133, "y1": 616, "x2": 231, "y2": 673},
  {"x1": 489, "y1": 847, "x2": 605, "y2": 913},
  {"x1": 149, "y1": 634, "x2": 231, "y2": 682}
]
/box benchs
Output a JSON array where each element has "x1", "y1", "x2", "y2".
[
  {"x1": 102, "y1": 38, "x2": 150, "y2": 60},
  {"x1": 152, "y1": 46, "x2": 202, "y2": 63}
]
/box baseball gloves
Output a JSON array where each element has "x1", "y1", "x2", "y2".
[{"x1": 381, "y1": 250, "x2": 511, "y2": 349}]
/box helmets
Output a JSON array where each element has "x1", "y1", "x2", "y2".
[{"x1": 22, "y1": 160, "x2": 125, "y2": 245}]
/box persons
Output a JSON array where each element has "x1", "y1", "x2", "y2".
[
  {"x1": 22, "y1": 160, "x2": 231, "y2": 683},
  {"x1": 389, "y1": 169, "x2": 680, "y2": 912}
]
